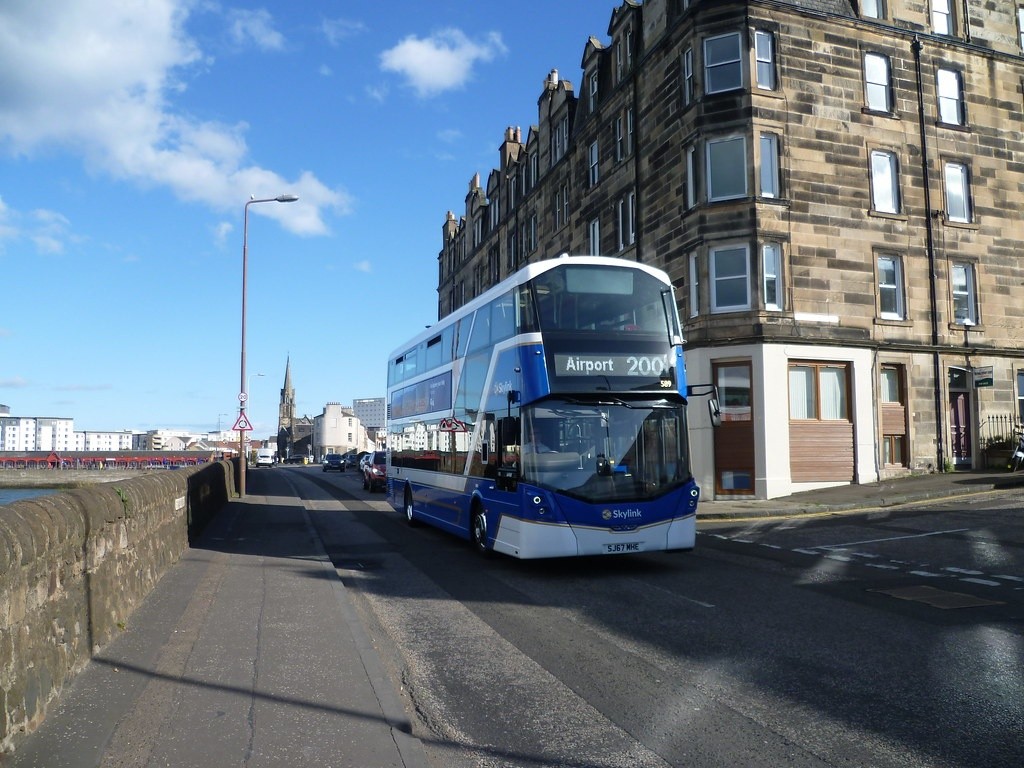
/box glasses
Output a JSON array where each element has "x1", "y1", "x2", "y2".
[{"x1": 529, "y1": 432, "x2": 542, "y2": 435}]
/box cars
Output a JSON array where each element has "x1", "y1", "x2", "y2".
[
  {"x1": 322, "y1": 453, "x2": 346, "y2": 472},
  {"x1": 255, "y1": 454, "x2": 272, "y2": 468},
  {"x1": 342, "y1": 450, "x2": 387, "y2": 494},
  {"x1": 283, "y1": 454, "x2": 305, "y2": 464}
]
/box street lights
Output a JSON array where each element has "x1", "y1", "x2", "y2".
[
  {"x1": 219, "y1": 413, "x2": 228, "y2": 431},
  {"x1": 240, "y1": 193, "x2": 300, "y2": 498},
  {"x1": 246, "y1": 374, "x2": 267, "y2": 459}
]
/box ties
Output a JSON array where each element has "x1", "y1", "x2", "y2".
[{"x1": 536, "y1": 445, "x2": 539, "y2": 454}]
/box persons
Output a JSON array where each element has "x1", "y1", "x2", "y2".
[{"x1": 525, "y1": 423, "x2": 550, "y2": 454}]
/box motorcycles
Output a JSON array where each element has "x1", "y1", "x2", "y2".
[{"x1": 1006, "y1": 425, "x2": 1024, "y2": 473}]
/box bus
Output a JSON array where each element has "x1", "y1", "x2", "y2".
[{"x1": 385, "y1": 254, "x2": 723, "y2": 561}]
